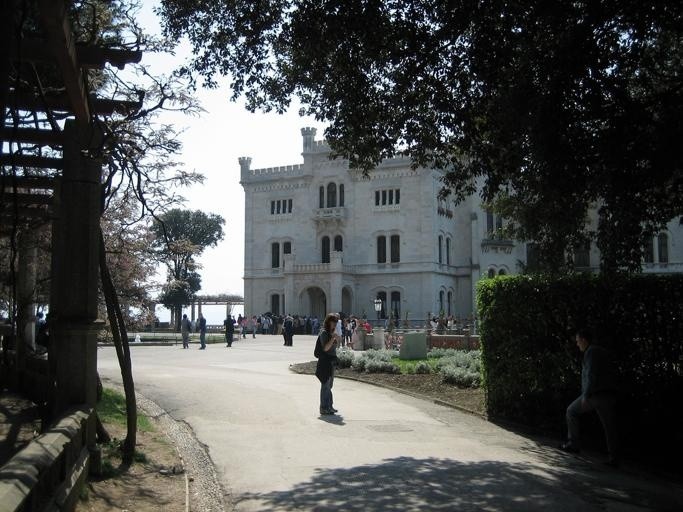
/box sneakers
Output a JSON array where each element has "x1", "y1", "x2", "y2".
[{"x1": 320, "y1": 406, "x2": 338, "y2": 415}]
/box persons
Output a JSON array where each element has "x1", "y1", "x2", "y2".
[
  {"x1": 557, "y1": 330, "x2": 619, "y2": 465},
  {"x1": 37, "y1": 311, "x2": 47, "y2": 326},
  {"x1": 180, "y1": 311, "x2": 480, "y2": 350},
  {"x1": 314, "y1": 312, "x2": 341, "y2": 414}
]
